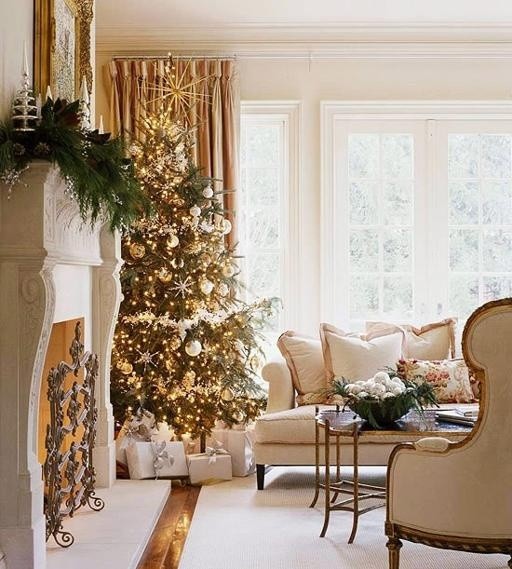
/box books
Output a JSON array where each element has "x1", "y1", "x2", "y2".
[
  {"x1": 455, "y1": 406, "x2": 479, "y2": 417},
  {"x1": 434, "y1": 415, "x2": 474, "y2": 427},
  {"x1": 435, "y1": 410, "x2": 478, "y2": 422}
]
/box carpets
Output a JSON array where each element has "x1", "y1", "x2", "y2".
[{"x1": 175, "y1": 463, "x2": 509, "y2": 568}]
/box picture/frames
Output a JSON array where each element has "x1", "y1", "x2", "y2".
[{"x1": 30, "y1": 0, "x2": 95, "y2": 124}]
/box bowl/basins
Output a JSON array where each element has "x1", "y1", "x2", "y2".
[{"x1": 347, "y1": 401, "x2": 412, "y2": 424}]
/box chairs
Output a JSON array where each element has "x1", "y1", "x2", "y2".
[{"x1": 383, "y1": 297, "x2": 512, "y2": 568}]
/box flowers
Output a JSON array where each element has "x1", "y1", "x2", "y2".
[{"x1": 296, "y1": 364, "x2": 442, "y2": 433}]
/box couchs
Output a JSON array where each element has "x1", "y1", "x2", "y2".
[{"x1": 251, "y1": 354, "x2": 484, "y2": 492}]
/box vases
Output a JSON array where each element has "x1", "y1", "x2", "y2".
[{"x1": 348, "y1": 399, "x2": 416, "y2": 431}]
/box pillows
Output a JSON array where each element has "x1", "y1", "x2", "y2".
[{"x1": 276, "y1": 315, "x2": 476, "y2": 407}]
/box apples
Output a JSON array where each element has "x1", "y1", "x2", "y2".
[{"x1": 344, "y1": 372, "x2": 413, "y2": 402}]
